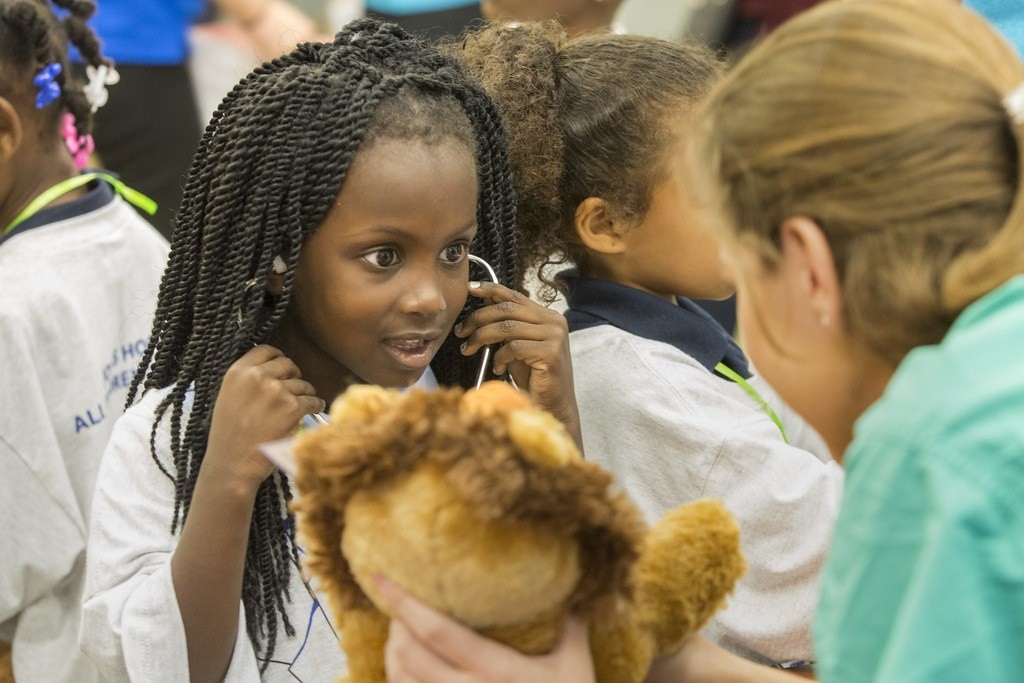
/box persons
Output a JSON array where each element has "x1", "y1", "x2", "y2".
[
  {"x1": 371, "y1": 0, "x2": 1024, "y2": 683},
  {"x1": 77, "y1": 17, "x2": 584, "y2": 683},
  {"x1": 0, "y1": 0, "x2": 172, "y2": 683},
  {"x1": 185, "y1": 0, "x2": 825, "y2": 315},
  {"x1": 450, "y1": 22, "x2": 842, "y2": 668}
]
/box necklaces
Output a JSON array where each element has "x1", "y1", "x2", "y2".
[
  {"x1": 715, "y1": 362, "x2": 787, "y2": 444},
  {"x1": 0, "y1": 172, "x2": 158, "y2": 239}
]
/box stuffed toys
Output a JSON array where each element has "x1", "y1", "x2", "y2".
[{"x1": 291, "y1": 380, "x2": 746, "y2": 683}]
